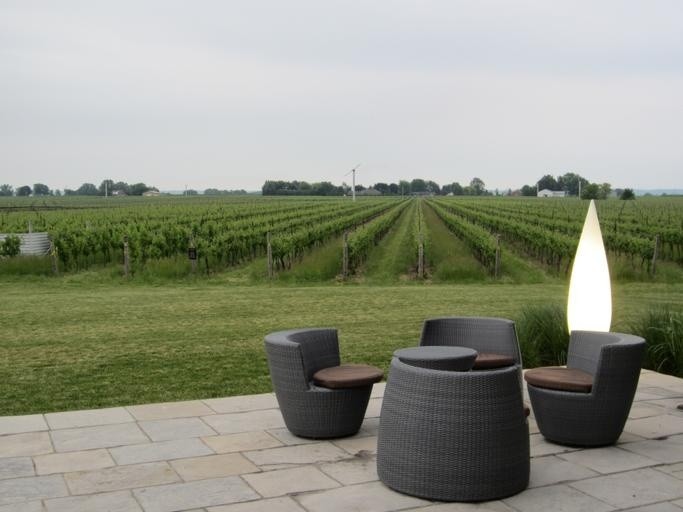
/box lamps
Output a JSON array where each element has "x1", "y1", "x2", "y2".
[{"x1": 565, "y1": 197, "x2": 613, "y2": 335}]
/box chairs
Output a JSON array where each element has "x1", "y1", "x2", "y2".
[
  {"x1": 375, "y1": 355, "x2": 529, "y2": 503},
  {"x1": 260, "y1": 325, "x2": 374, "y2": 438},
  {"x1": 526, "y1": 329, "x2": 647, "y2": 448},
  {"x1": 417, "y1": 316, "x2": 522, "y2": 371}
]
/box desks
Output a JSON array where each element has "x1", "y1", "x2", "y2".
[{"x1": 392, "y1": 346, "x2": 478, "y2": 371}]
